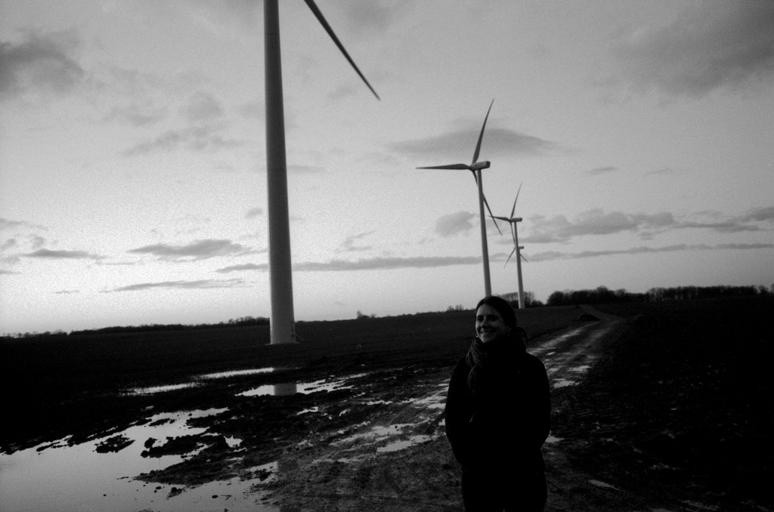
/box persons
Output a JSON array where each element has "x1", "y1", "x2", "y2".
[{"x1": 445, "y1": 298, "x2": 553, "y2": 512}]
